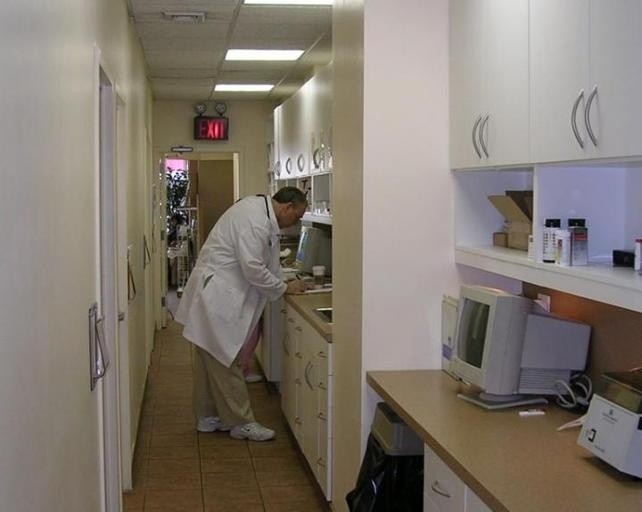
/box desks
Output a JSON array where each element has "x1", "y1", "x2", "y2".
[{"x1": 365, "y1": 369, "x2": 642, "y2": 512}]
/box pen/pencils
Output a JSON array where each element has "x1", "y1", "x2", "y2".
[{"x1": 296, "y1": 275, "x2": 300, "y2": 280}]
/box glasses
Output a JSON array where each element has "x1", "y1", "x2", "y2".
[{"x1": 294, "y1": 216, "x2": 303, "y2": 225}]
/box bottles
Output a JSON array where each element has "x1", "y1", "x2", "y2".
[{"x1": 541, "y1": 218, "x2": 589, "y2": 268}]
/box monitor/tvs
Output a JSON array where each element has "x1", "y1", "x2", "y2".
[
  {"x1": 450, "y1": 284, "x2": 591, "y2": 410},
  {"x1": 294, "y1": 226, "x2": 331, "y2": 282}
]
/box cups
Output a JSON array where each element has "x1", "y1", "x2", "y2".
[
  {"x1": 313, "y1": 265, "x2": 328, "y2": 289},
  {"x1": 314, "y1": 200, "x2": 329, "y2": 214}
]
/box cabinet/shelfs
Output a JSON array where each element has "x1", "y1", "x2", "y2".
[
  {"x1": 272, "y1": 59, "x2": 333, "y2": 182},
  {"x1": 533, "y1": 0, "x2": 641, "y2": 166},
  {"x1": 448, "y1": 0, "x2": 533, "y2": 168},
  {"x1": 253, "y1": 290, "x2": 333, "y2": 502}
]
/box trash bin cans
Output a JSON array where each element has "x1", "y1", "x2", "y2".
[{"x1": 345, "y1": 402, "x2": 423, "y2": 512}]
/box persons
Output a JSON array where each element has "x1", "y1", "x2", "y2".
[
  {"x1": 238, "y1": 316, "x2": 265, "y2": 384},
  {"x1": 173, "y1": 187, "x2": 308, "y2": 441}
]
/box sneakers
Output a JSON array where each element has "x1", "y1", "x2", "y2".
[
  {"x1": 243, "y1": 374, "x2": 262, "y2": 384},
  {"x1": 196, "y1": 415, "x2": 232, "y2": 432},
  {"x1": 230, "y1": 421, "x2": 276, "y2": 442}
]
